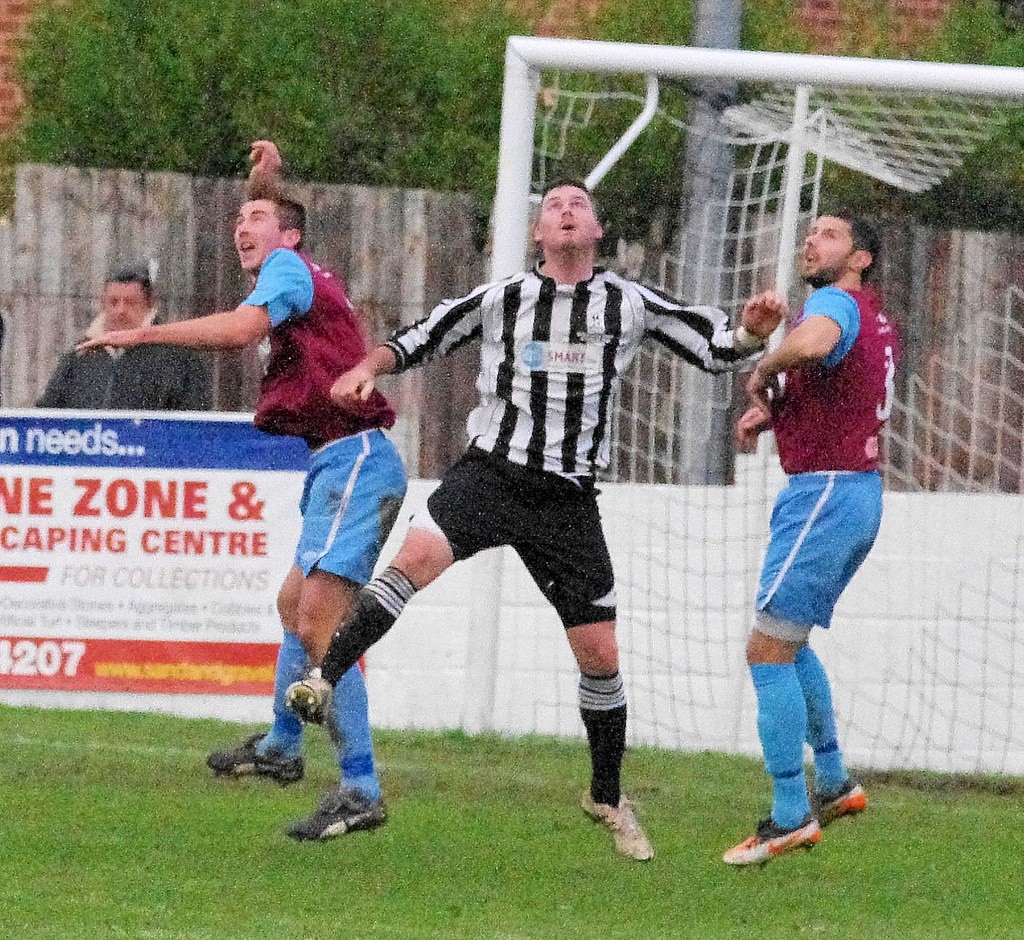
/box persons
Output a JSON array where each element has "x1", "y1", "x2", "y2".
[
  {"x1": 77, "y1": 137, "x2": 408, "y2": 842},
  {"x1": 281, "y1": 179, "x2": 787, "y2": 863},
  {"x1": 36, "y1": 271, "x2": 215, "y2": 415},
  {"x1": 720, "y1": 204, "x2": 895, "y2": 868}
]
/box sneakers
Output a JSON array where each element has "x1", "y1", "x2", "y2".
[
  {"x1": 723, "y1": 811, "x2": 822, "y2": 867},
  {"x1": 287, "y1": 791, "x2": 384, "y2": 842},
  {"x1": 807, "y1": 776, "x2": 866, "y2": 828},
  {"x1": 207, "y1": 732, "x2": 304, "y2": 784},
  {"x1": 286, "y1": 668, "x2": 334, "y2": 726},
  {"x1": 580, "y1": 787, "x2": 655, "y2": 861}
]
